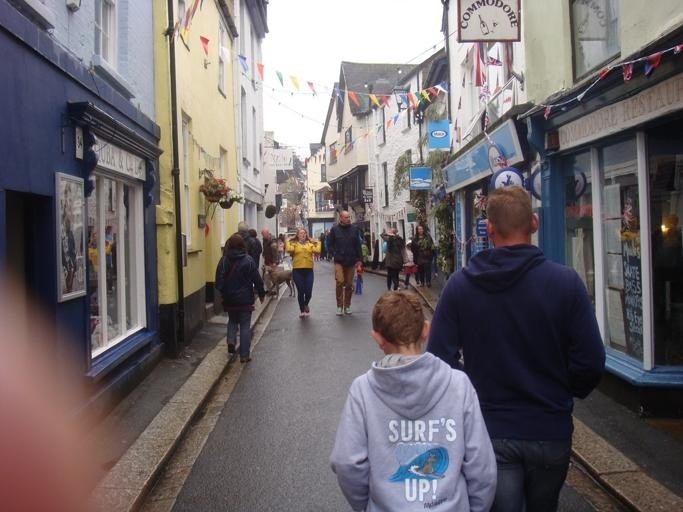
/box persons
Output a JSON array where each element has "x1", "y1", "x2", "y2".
[
  {"x1": 354, "y1": 227, "x2": 370, "y2": 275},
  {"x1": 331, "y1": 287, "x2": 499, "y2": 512},
  {"x1": 88, "y1": 225, "x2": 117, "y2": 349},
  {"x1": 232, "y1": 222, "x2": 333, "y2": 320},
  {"x1": 429, "y1": 185, "x2": 605, "y2": 510},
  {"x1": 326, "y1": 211, "x2": 362, "y2": 315},
  {"x1": 0, "y1": 267, "x2": 121, "y2": 512},
  {"x1": 379, "y1": 225, "x2": 436, "y2": 293},
  {"x1": 214, "y1": 234, "x2": 265, "y2": 363}
]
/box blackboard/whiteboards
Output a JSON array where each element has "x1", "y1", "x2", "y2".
[{"x1": 619, "y1": 184, "x2": 643, "y2": 363}]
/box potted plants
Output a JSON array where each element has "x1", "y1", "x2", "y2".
[{"x1": 218, "y1": 193, "x2": 243, "y2": 209}]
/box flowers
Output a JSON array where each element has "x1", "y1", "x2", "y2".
[{"x1": 200, "y1": 177, "x2": 230, "y2": 203}]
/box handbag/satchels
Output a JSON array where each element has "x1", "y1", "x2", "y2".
[
  {"x1": 355, "y1": 274, "x2": 363, "y2": 296},
  {"x1": 217, "y1": 266, "x2": 226, "y2": 291}
]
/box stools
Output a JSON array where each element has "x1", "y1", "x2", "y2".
[{"x1": 655, "y1": 266, "x2": 679, "y2": 323}]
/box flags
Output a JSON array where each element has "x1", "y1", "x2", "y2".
[{"x1": 471, "y1": 41, "x2": 486, "y2": 87}]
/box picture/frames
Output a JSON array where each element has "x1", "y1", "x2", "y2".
[{"x1": 53, "y1": 171, "x2": 86, "y2": 304}]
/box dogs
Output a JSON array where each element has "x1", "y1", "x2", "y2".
[{"x1": 265, "y1": 264, "x2": 296, "y2": 299}]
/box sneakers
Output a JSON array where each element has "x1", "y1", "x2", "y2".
[
  {"x1": 420, "y1": 281, "x2": 424, "y2": 287},
  {"x1": 336, "y1": 305, "x2": 344, "y2": 316},
  {"x1": 344, "y1": 305, "x2": 353, "y2": 315},
  {"x1": 404, "y1": 284, "x2": 409, "y2": 289},
  {"x1": 303, "y1": 303, "x2": 310, "y2": 313},
  {"x1": 426, "y1": 281, "x2": 431, "y2": 288},
  {"x1": 416, "y1": 280, "x2": 420, "y2": 284},
  {"x1": 228, "y1": 343, "x2": 236, "y2": 354},
  {"x1": 299, "y1": 311, "x2": 305, "y2": 318},
  {"x1": 240, "y1": 354, "x2": 252, "y2": 364}
]
